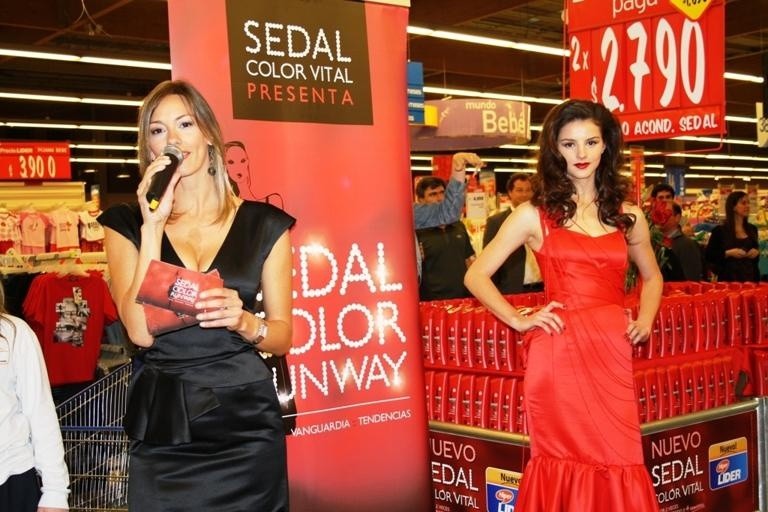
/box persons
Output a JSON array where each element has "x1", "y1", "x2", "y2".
[
  {"x1": 412, "y1": 150, "x2": 544, "y2": 299},
  {"x1": 1, "y1": 275, "x2": 72, "y2": 512},
  {"x1": 643, "y1": 183, "x2": 759, "y2": 281},
  {"x1": 97, "y1": 78, "x2": 297, "y2": 512},
  {"x1": 460, "y1": 100, "x2": 665, "y2": 512}
]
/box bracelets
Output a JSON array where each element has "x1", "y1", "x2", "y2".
[{"x1": 242, "y1": 316, "x2": 268, "y2": 345}]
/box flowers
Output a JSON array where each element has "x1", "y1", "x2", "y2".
[{"x1": 640, "y1": 185, "x2": 674, "y2": 249}]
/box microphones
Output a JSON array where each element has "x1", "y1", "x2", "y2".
[{"x1": 146, "y1": 144, "x2": 183, "y2": 211}]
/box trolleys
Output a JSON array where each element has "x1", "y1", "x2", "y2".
[{"x1": 53, "y1": 354, "x2": 131, "y2": 512}]
